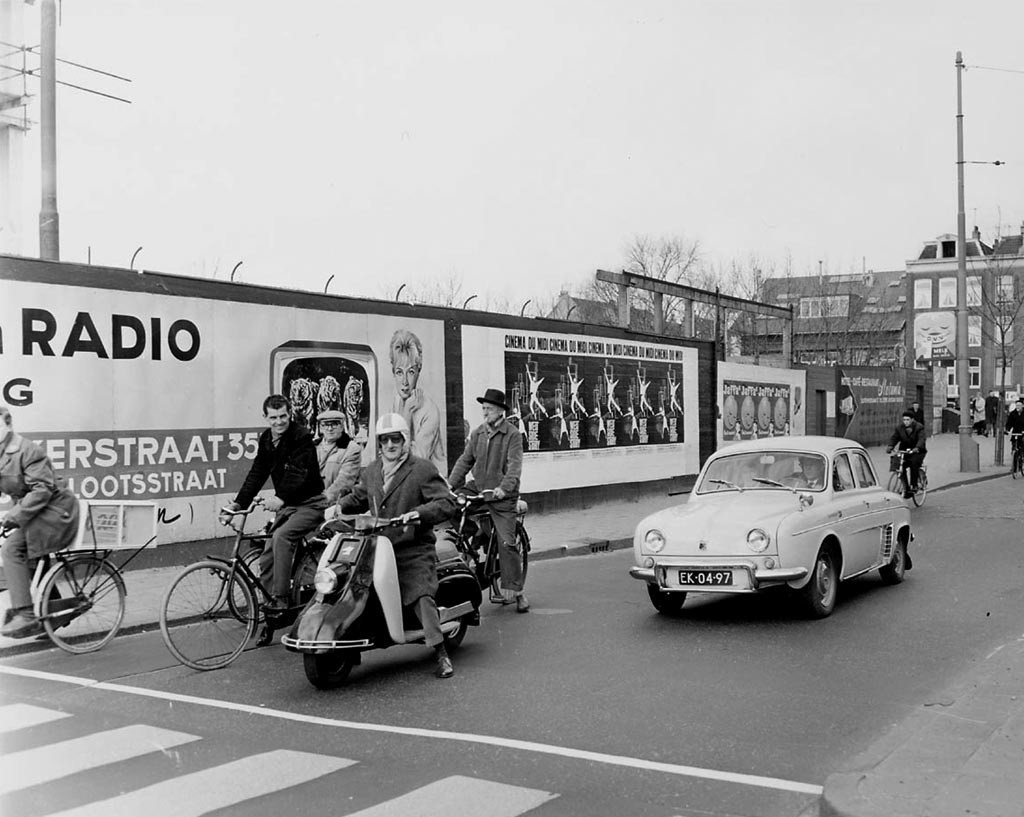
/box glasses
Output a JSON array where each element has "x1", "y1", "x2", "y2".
[
  {"x1": 320, "y1": 422, "x2": 341, "y2": 428},
  {"x1": 381, "y1": 436, "x2": 402, "y2": 443}
]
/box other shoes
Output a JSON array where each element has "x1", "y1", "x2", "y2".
[
  {"x1": 34, "y1": 620, "x2": 70, "y2": 640},
  {"x1": 435, "y1": 657, "x2": 454, "y2": 678},
  {"x1": 470, "y1": 531, "x2": 489, "y2": 551},
  {"x1": 993, "y1": 432, "x2": 995, "y2": 437},
  {"x1": 912, "y1": 485, "x2": 919, "y2": 492},
  {"x1": 255, "y1": 625, "x2": 276, "y2": 646},
  {"x1": 899, "y1": 486, "x2": 911, "y2": 495},
  {"x1": 0, "y1": 613, "x2": 40, "y2": 636},
  {"x1": 260, "y1": 595, "x2": 289, "y2": 612},
  {"x1": 983, "y1": 432, "x2": 988, "y2": 437},
  {"x1": 515, "y1": 593, "x2": 530, "y2": 611},
  {"x1": 1010, "y1": 469, "x2": 1017, "y2": 473}
]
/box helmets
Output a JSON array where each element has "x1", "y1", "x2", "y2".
[{"x1": 375, "y1": 412, "x2": 409, "y2": 434}]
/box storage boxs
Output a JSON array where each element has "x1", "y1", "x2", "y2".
[{"x1": 78, "y1": 498, "x2": 158, "y2": 551}]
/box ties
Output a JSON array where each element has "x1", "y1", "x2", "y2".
[{"x1": 807, "y1": 482, "x2": 814, "y2": 488}]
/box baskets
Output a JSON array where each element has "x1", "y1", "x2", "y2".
[{"x1": 890, "y1": 455, "x2": 902, "y2": 472}]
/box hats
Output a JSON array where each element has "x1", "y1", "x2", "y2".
[
  {"x1": 317, "y1": 410, "x2": 345, "y2": 422},
  {"x1": 476, "y1": 388, "x2": 510, "y2": 412}
]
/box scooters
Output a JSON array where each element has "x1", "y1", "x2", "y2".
[{"x1": 280, "y1": 485, "x2": 483, "y2": 691}]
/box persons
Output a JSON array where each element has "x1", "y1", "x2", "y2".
[
  {"x1": 0, "y1": 405, "x2": 80, "y2": 641},
  {"x1": 785, "y1": 457, "x2": 842, "y2": 492},
  {"x1": 445, "y1": 389, "x2": 530, "y2": 612},
  {"x1": 218, "y1": 394, "x2": 325, "y2": 647},
  {"x1": 885, "y1": 411, "x2": 928, "y2": 499},
  {"x1": 323, "y1": 412, "x2": 459, "y2": 678},
  {"x1": 907, "y1": 402, "x2": 924, "y2": 426},
  {"x1": 507, "y1": 363, "x2": 683, "y2": 445},
  {"x1": 953, "y1": 389, "x2": 998, "y2": 437},
  {"x1": 312, "y1": 411, "x2": 362, "y2": 509},
  {"x1": 1004, "y1": 400, "x2": 1024, "y2": 471},
  {"x1": 388, "y1": 330, "x2": 449, "y2": 479}
]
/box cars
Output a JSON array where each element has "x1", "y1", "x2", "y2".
[{"x1": 629, "y1": 435, "x2": 915, "y2": 618}]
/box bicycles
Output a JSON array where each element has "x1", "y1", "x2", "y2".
[
  {"x1": 886, "y1": 448, "x2": 929, "y2": 507},
  {"x1": 447, "y1": 489, "x2": 532, "y2": 607},
  {"x1": 158, "y1": 494, "x2": 349, "y2": 672},
  {"x1": 0, "y1": 525, "x2": 130, "y2": 654},
  {"x1": 1003, "y1": 430, "x2": 1024, "y2": 479}
]
